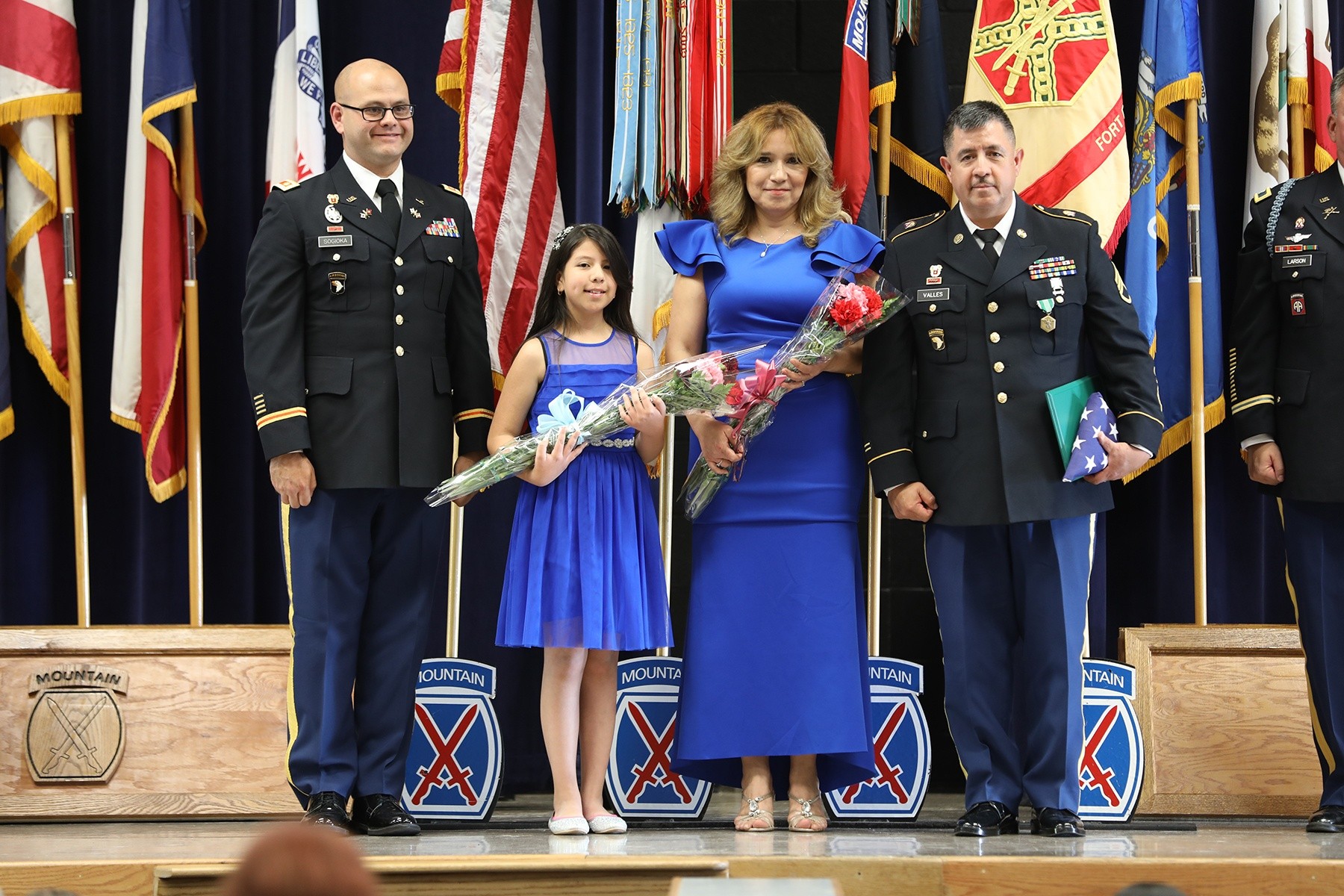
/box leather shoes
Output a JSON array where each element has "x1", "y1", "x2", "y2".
[
  {"x1": 300, "y1": 791, "x2": 349, "y2": 833},
  {"x1": 1029, "y1": 806, "x2": 1086, "y2": 838},
  {"x1": 350, "y1": 794, "x2": 421, "y2": 836},
  {"x1": 954, "y1": 800, "x2": 1019, "y2": 836},
  {"x1": 1306, "y1": 805, "x2": 1344, "y2": 832}
]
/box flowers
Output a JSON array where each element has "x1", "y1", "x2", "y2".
[
  {"x1": 677, "y1": 277, "x2": 903, "y2": 517},
  {"x1": 433, "y1": 343, "x2": 743, "y2": 500}
]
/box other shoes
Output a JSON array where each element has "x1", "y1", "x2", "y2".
[
  {"x1": 587, "y1": 813, "x2": 628, "y2": 833},
  {"x1": 548, "y1": 809, "x2": 590, "y2": 835}
]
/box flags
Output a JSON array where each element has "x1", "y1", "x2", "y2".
[
  {"x1": 110, "y1": 0, "x2": 204, "y2": 505},
  {"x1": 0, "y1": 0, "x2": 88, "y2": 440},
  {"x1": 1242, "y1": 0, "x2": 1335, "y2": 254},
  {"x1": 964, "y1": 0, "x2": 1230, "y2": 486},
  {"x1": 628, "y1": 200, "x2": 692, "y2": 375},
  {"x1": 264, "y1": 0, "x2": 328, "y2": 204},
  {"x1": 433, "y1": 0, "x2": 566, "y2": 403},
  {"x1": 833, "y1": 0, "x2": 951, "y2": 249}
]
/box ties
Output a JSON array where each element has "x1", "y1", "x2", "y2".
[
  {"x1": 974, "y1": 229, "x2": 1000, "y2": 270},
  {"x1": 377, "y1": 179, "x2": 403, "y2": 244}
]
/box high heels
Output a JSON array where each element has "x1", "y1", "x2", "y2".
[
  {"x1": 733, "y1": 782, "x2": 775, "y2": 831},
  {"x1": 788, "y1": 776, "x2": 828, "y2": 830}
]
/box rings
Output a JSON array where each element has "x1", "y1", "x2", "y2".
[
  {"x1": 802, "y1": 380, "x2": 805, "y2": 387},
  {"x1": 716, "y1": 460, "x2": 723, "y2": 467}
]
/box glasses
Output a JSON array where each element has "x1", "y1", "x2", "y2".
[{"x1": 338, "y1": 103, "x2": 417, "y2": 122}]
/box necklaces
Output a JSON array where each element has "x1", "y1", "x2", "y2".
[{"x1": 754, "y1": 216, "x2": 800, "y2": 257}]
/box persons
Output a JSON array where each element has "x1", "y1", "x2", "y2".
[
  {"x1": 484, "y1": 226, "x2": 674, "y2": 837},
  {"x1": 238, "y1": 59, "x2": 500, "y2": 830},
  {"x1": 666, "y1": 103, "x2": 883, "y2": 836},
  {"x1": 860, "y1": 99, "x2": 1164, "y2": 836},
  {"x1": 1226, "y1": 70, "x2": 1344, "y2": 833}
]
